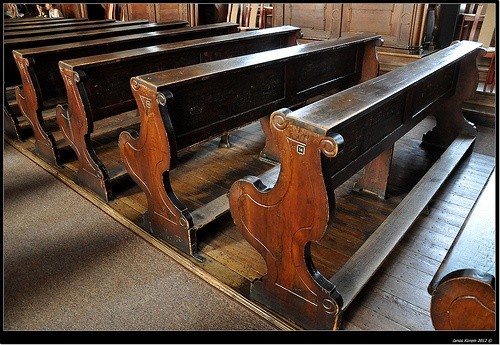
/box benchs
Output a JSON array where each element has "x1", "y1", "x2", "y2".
[{"x1": 2, "y1": 1, "x2": 495, "y2": 331}]
